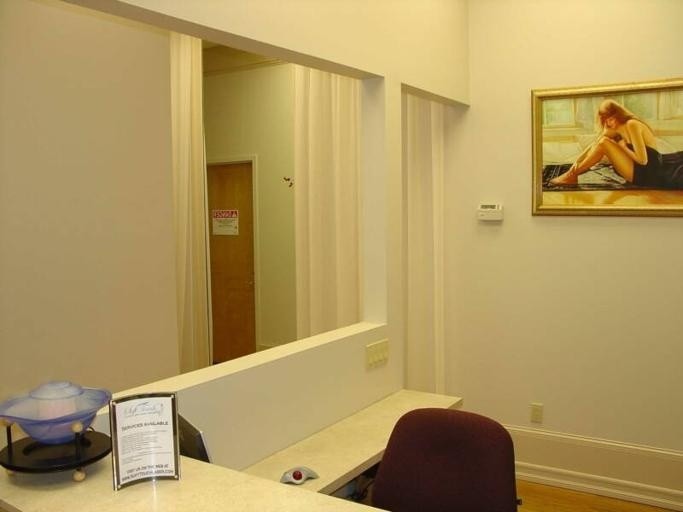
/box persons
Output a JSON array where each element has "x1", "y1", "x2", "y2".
[{"x1": 547, "y1": 98, "x2": 663, "y2": 189}]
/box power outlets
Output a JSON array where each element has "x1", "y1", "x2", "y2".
[{"x1": 530, "y1": 403, "x2": 544, "y2": 423}]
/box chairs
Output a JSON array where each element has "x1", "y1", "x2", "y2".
[{"x1": 357, "y1": 408, "x2": 520, "y2": 511}]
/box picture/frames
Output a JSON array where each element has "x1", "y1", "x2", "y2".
[{"x1": 531, "y1": 77, "x2": 682, "y2": 217}]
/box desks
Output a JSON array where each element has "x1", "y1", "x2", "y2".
[{"x1": 0, "y1": 319, "x2": 461, "y2": 511}]
